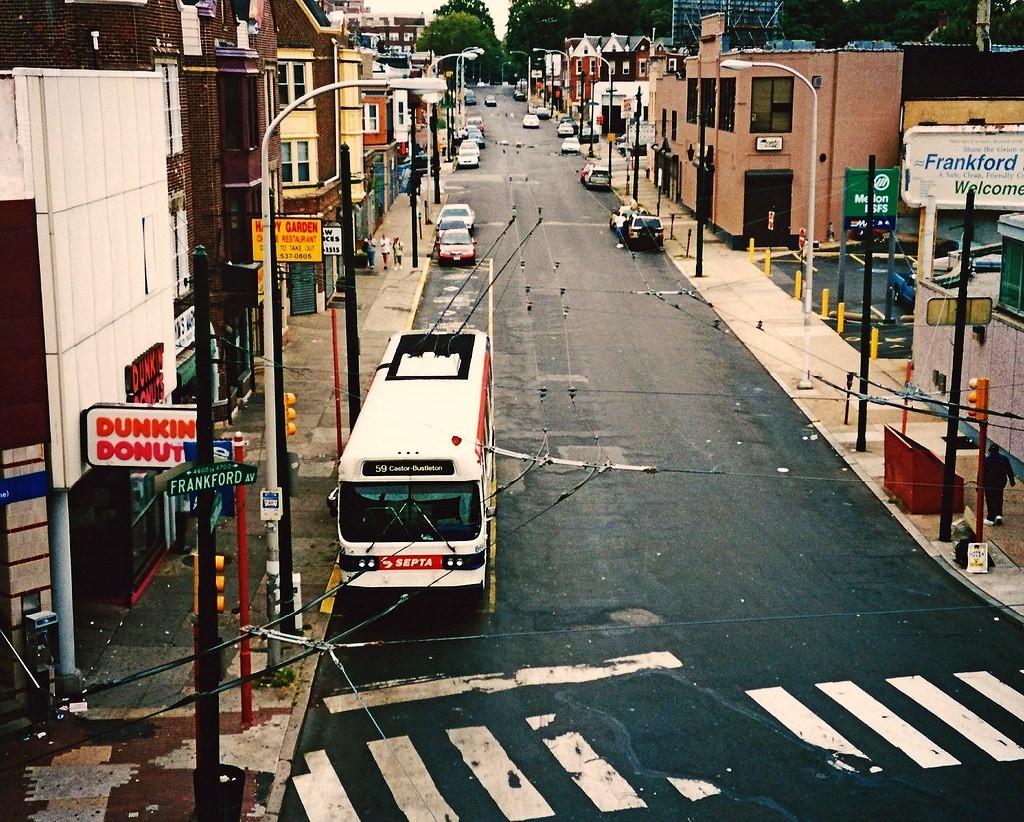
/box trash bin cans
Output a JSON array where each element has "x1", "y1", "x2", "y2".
[
  {"x1": 884, "y1": 424, "x2": 966, "y2": 514},
  {"x1": 275, "y1": 572, "x2": 302, "y2": 629}
]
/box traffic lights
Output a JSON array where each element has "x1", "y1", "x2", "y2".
[
  {"x1": 193, "y1": 552, "x2": 225, "y2": 614},
  {"x1": 969, "y1": 377, "x2": 989, "y2": 420},
  {"x1": 284, "y1": 392, "x2": 297, "y2": 438}
]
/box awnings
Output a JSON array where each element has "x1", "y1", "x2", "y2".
[{"x1": 176, "y1": 340, "x2": 216, "y2": 386}]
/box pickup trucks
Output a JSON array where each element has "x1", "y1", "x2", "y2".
[{"x1": 891, "y1": 266, "x2": 918, "y2": 317}]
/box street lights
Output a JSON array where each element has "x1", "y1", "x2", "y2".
[
  {"x1": 546, "y1": 50, "x2": 570, "y2": 116},
  {"x1": 455, "y1": 48, "x2": 486, "y2": 139},
  {"x1": 720, "y1": 58, "x2": 817, "y2": 326},
  {"x1": 426, "y1": 51, "x2": 478, "y2": 223},
  {"x1": 460, "y1": 46, "x2": 480, "y2": 128},
  {"x1": 263, "y1": 77, "x2": 448, "y2": 676},
  {"x1": 533, "y1": 48, "x2": 553, "y2": 115},
  {"x1": 581, "y1": 54, "x2": 612, "y2": 169},
  {"x1": 510, "y1": 51, "x2": 531, "y2": 101}
]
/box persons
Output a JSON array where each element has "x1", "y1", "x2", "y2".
[
  {"x1": 441, "y1": 137, "x2": 446, "y2": 156},
  {"x1": 453, "y1": 127, "x2": 469, "y2": 146},
  {"x1": 451, "y1": 144, "x2": 456, "y2": 162},
  {"x1": 364, "y1": 233, "x2": 377, "y2": 269},
  {"x1": 392, "y1": 237, "x2": 405, "y2": 271},
  {"x1": 983, "y1": 443, "x2": 1015, "y2": 526},
  {"x1": 613, "y1": 211, "x2": 626, "y2": 247},
  {"x1": 380, "y1": 233, "x2": 392, "y2": 270}
]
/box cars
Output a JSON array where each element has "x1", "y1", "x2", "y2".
[
  {"x1": 436, "y1": 228, "x2": 477, "y2": 265},
  {"x1": 580, "y1": 162, "x2": 612, "y2": 189},
  {"x1": 464, "y1": 94, "x2": 477, "y2": 106},
  {"x1": 522, "y1": 115, "x2": 540, "y2": 129},
  {"x1": 610, "y1": 204, "x2": 665, "y2": 250},
  {"x1": 561, "y1": 137, "x2": 581, "y2": 153},
  {"x1": 435, "y1": 217, "x2": 469, "y2": 243},
  {"x1": 557, "y1": 117, "x2": 579, "y2": 137},
  {"x1": 484, "y1": 95, "x2": 497, "y2": 107},
  {"x1": 453, "y1": 114, "x2": 486, "y2": 169},
  {"x1": 527, "y1": 101, "x2": 550, "y2": 120},
  {"x1": 578, "y1": 127, "x2": 600, "y2": 145},
  {"x1": 436, "y1": 203, "x2": 476, "y2": 230}
]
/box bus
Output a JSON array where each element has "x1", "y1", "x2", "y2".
[{"x1": 326, "y1": 207, "x2": 544, "y2": 596}]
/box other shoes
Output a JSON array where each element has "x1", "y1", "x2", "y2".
[
  {"x1": 995, "y1": 515, "x2": 1003, "y2": 525},
  {"x1": 983, "y1": 519, "x2": 994, "y2": 526}
]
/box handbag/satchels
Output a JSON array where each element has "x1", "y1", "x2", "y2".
[{"x1": 966, "y1": 543, "x2": 988, "y2": 573}]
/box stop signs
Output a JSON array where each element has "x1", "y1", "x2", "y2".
[
  {"x1": 767, "y1": 212, "x2": 773, "y2": 228},
  {"x1": 799, "y1": 229, "x2": 805, "y2": 248}
]
